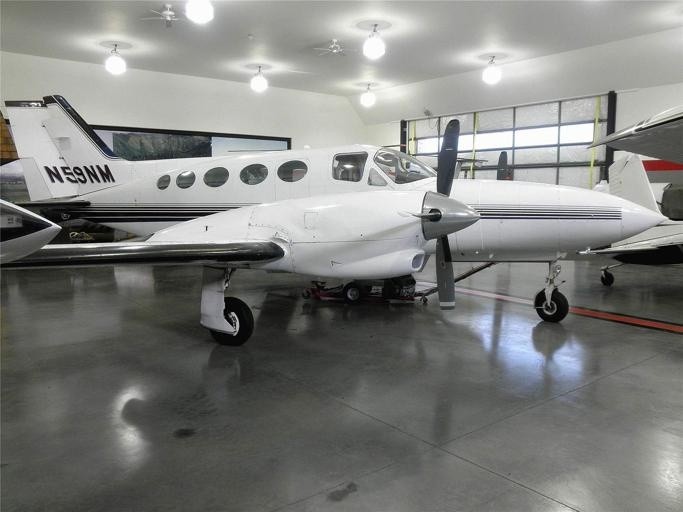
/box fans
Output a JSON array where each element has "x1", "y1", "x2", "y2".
[{"x1": 139, "y1": 4, "x2": 351, "y2": 58}]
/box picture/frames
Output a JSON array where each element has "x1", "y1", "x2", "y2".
[{"x1": 4, "y1": 119, "x2": 291, "y2": 161}]
